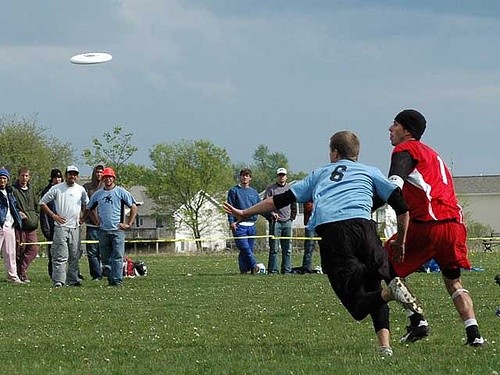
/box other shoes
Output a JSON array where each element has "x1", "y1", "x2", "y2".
[
  {"x1": 53, "y1": 281, "x2": 63, "y2": 287},
  {"x1": 4, "y1": 278, "x2": 24, "y2": 284},
  {"x1": 109, "y1": 281, "x2": 124, "y2": 286},
  {"x1": 93, "y1": 276, "x2": 101, "y2": 281},
  {"x1": 78, "y1": 273, "x2": 83, "y2": 281},
  {"x1": 23, "y1": 280, "x2": 30, "y2": 283},
  {"x1": 74, "y1": 282, "x2": 82, "y2": 286}
]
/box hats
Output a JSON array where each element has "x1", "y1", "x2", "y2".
[
  {"x1": 51, "y1": 169, "x2": 62, "y2": 179},
  {"x1": 276, "y1": 168, "x2": 288, "y2": 176},
  {"x1": 100, "y1": 168, "x2": 116, "y2": 180},
  {"x1": 395, "y1": 110, "x2": 426, "y2": 141},
  {"x1": 0, "y1": 165, "x2": 10, "y2": 181},
  {"x1": 65, "y1": 165, "x2": 80, "y2": 174}
]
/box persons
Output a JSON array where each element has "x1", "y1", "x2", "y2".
[
  {"x1": 227, "y1": 168, "x2": 278, "y2": 276},
  {"x1": 39, "y1": 168, "x2": 64, "y2": 279},
  {"x1": 222, "y1": 131, "x2": 425, "y2": 357},
  {"x1": 302, "y1": 200, "x2": 316, "y2": 269},
  {"x1": 11, "y1": 167, "x2": 39, "y2": 282},
  {"x1": 263, "y1": 167, "x2": 299, "y2": 275},
  {"x1": 384, "y1": 109, "x2": 487, "y2": 348},
  {"x1": 0, "y1": 166, "x2": 25, "y2": 284},
  {"x1": 38, "y1": 165, "x2": 91, "y2": 287},
  {"x1": 81, "y1": 165, "x2": 105, "y2": 281},
  {"x1": 85, "y1": 168, "x2": 139, "y2": 286}
]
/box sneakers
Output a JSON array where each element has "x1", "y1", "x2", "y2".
[
  {"x1": 400, "y1": 321, "x2": 430, "y2": 344},
  {"x1": 379, "y1": 346, "x2": 393, "y2": 357},
  {"x1": 462, "y1": 336, "x2": 486, "y2": 347},
  {"x1": 387, "y1": 276, "x2": 423, "y2": 315}
]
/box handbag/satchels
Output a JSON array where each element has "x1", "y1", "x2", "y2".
[{"x1": 122, "y1": 257, "x2": 147, "y2": 278}]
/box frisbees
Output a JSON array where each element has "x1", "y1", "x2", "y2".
[{"x1": 69, "y1": 52, "x2": 113, "y2": 64}]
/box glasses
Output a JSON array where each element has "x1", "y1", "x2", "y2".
[{"x1": 53, "y1": 175, "x2": 62, "y2": 178}]
